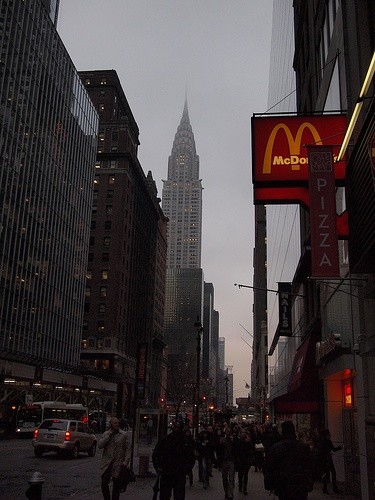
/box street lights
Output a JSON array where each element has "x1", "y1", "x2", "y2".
[
  {"x1": 224, "y1": 369, "x2": 230, "y2": 406},
  {"x1": 194, "y1": 315, "x2": 203, "y2": 437}
]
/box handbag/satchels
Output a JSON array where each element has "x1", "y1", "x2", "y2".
[
  {"x1": 302, "y1": 472, "x2": 314, "y2": 492},
  {"x1": 118, "y1": 464, "x2": 132, "y2": 492}
]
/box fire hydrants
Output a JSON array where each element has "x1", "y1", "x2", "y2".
[{"x1": 25, "y1": 471, "x2": 46, "y2": 500}]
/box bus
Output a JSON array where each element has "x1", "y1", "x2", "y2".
[{"x1": 16, "y1": 402, "x2": 89, "y2": 436}]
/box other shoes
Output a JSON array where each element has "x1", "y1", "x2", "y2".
[
  {"x1": 224, "y1": 493, "x2": 234, "y2": 500},
  {"x1": 203, "y1": 483, "x2": 208, "y2": 489},
  {"x1": 322, "y1": 490, "x2": 329, "y2": 495},
  {"x1": 334, "y1": 490, "x2": 344, "y2": 493},
  {"x1": 243, "y1": 486, "x2": 247, "y2": 495},
  {"x1": 239, "y1": 484, "x2": 243, "y2": 492}
]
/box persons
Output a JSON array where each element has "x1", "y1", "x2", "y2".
[
  {"x1": 89, "y1": 420, "x2": 97, "y2": 434},
  {"x1": 124, "y1": 416, "x2": 344, "y2": 500},
  {"x1": 97, "y1": 416, "x2": 131, "y2": 500}
]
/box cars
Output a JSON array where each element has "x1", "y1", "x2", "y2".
[
  {"x1": 32, "y1": 418, "x2": 97, "y2": 458},
  {"x1": 88, "y1": 412, "x2": 112, "y2": 432}
]
